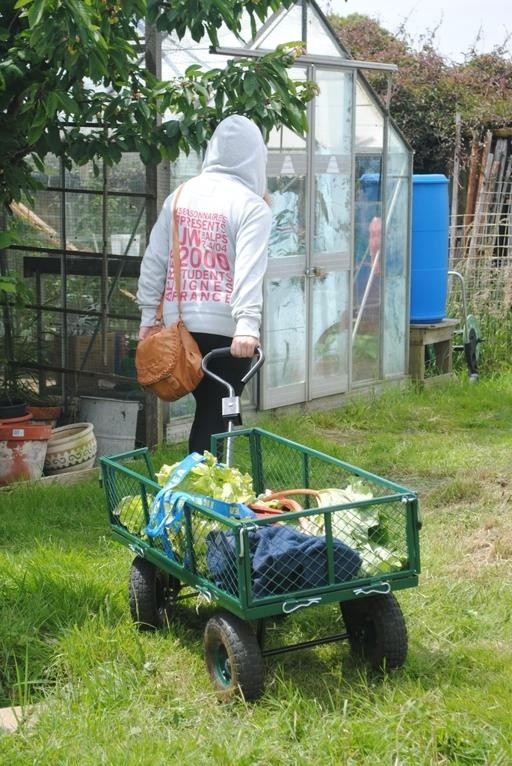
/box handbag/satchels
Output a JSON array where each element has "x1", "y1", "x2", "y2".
[{"x1": 135, "y1": 320, "x2": 204, "y2": 400}]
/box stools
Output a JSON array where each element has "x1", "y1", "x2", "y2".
[{"x1": 408, "y1": 318, "x2": 459, "y2": 387}]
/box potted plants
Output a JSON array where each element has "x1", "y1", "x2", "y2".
[{"x1": 0, "y1": 272, "x2": 39, "y2": 418}]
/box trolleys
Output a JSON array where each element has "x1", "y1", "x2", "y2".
[
  {"x1": 448, "y1": 270, "x2": 486, "y2": 387},
  {"x1": 98, "y1": 345, "x2": 423, "y2": 702}
]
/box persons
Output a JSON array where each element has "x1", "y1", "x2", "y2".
[{"x1": 136, "y1": 114, "x2": 272, "y2": 462}]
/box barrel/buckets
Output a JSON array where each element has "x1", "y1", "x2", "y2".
[
  {"x1": 79, "y1": 395, "x2": 143, "y2": 468},
  {"x1": 358, "y1": 173, "x2": 450, "y2": 325}
]
